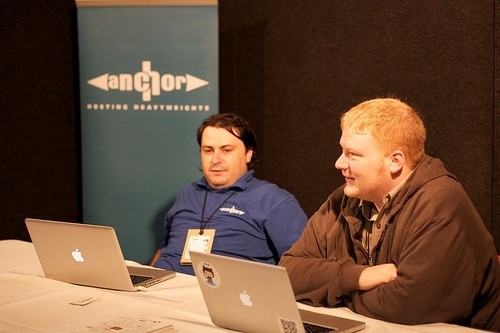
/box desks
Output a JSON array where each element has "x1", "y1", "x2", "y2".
[{"x1": 0, "y1": 239, "x2": 497, "y2": 333}]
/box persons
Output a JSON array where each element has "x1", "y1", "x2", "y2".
[
  {"x1": 278, "y1": 97, "x2": 500, "y2": 333},
  {"x1": 150, "y1": 112, "x2": 310, "y2": 277}
]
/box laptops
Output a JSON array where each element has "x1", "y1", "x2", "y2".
[
  {"x1": 24, "y1": 218, "x2": 176, "y2": 292},
  {"x1": 189, "y1": 249, "x2": 366, "y2": 333}
]
